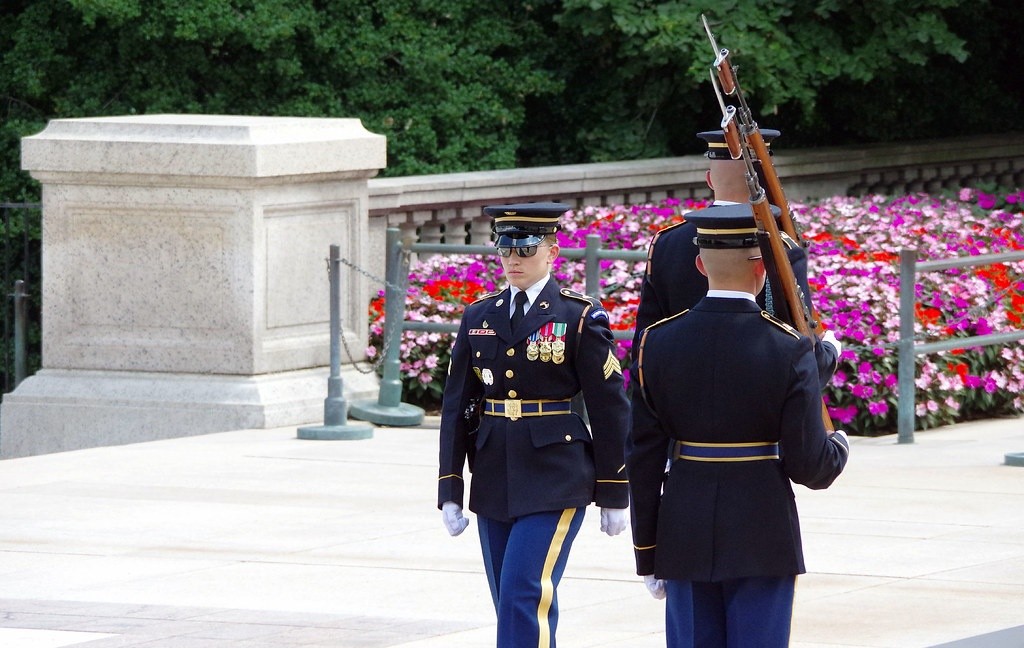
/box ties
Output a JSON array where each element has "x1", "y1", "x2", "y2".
[{"x1": 510, "y1": 291, "x2": 528, "y2": 335}]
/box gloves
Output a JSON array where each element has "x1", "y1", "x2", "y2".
[
  {"x1": 825, "y1": 429, "x2": 849, "y2": 445},
  {"x1": 644, "y1": 574, "x2": 667, "y2": 600},
  {"x1": 600, "y1": 505, "x2": 627, "y2": 536},
  {"x1": 822, "y1": 329, "x2": 842, "y2": 357},
  {"x1": 442, "y1": 500, "x2": 469, "y2": 536}
]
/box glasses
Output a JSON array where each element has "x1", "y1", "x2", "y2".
[{"x1": 496, "y1": 244, "x2": 558, "y2": 258}]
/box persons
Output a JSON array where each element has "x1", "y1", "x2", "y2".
[
  {"x1": 629, "y1": 130, "x2": 842, "y2": 391},
  {"x1": 623, "y1": 204, "x2": 849, "y2": 648},
  {"x1": 438, "y1": 203, "x2": 631, "y2": 648}
]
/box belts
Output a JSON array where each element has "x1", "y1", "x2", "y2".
[
  {"x1": 484, "y1": 397, "x2": 572, "y2": 421},
  {"x1": 668, "y1": 436, "x2": 779, "y2": 462}
]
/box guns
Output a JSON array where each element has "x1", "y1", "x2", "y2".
[
  {"x1": 706, "y1": 68, "x2": 835, "y2": 434},
  {"x1": 696, "y1": 13, "x2": 806, "y2": 247}
]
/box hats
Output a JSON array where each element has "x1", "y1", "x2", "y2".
[
  {"x1": 483, "y1": 203, "x2": 572, "y2": 248},
  {"x1": 684, "y1": 201, "x2": 782, "y2": 249},
  {"x1": 697, "y1": 129, "x2": 782, "y2": 159}
]
